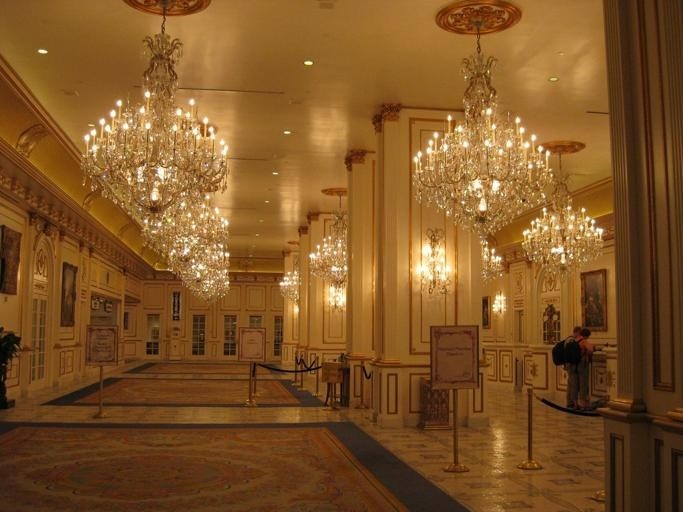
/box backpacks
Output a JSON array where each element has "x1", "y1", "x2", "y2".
[
  {"x1": 552, "y1": 335, "x2": 572, "y2": 366},
  {"x1": 566, "y1": 339, "x2": 583, "y2": 366}
]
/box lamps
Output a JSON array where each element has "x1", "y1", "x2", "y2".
[
  {"x1": 414, "y1": 228, "x2": 455, "y2": 297},
  {"x1": 308, "y1": 186, "x2": 352, "y2": 311},
  {"x1": 522, "y1": 139, "x2": 605, "y2": 282},
  {"x1": 278, "y1": 241, "x2": 302, "y2": 305},
  {"x1": 411, "y1": 0, "x2": 554, "y2": 245},
  {"x1": 478, "y1": 239, "x2": 504, "y2": 288},
  {"x1": 75, "y1": 0, "x2": 232, "y2": 300},
  {"x1": 494, "y1": 292, "x2": 507, "y2": 315}
]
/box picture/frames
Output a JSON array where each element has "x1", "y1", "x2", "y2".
[
  {"x1": 579, "y1": 269, "x2": 609, "y2": 332},
  {"x1": 482, "y1": 295, "x2": 491, "y2": 330}
]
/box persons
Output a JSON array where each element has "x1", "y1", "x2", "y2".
[
  {"x1": 562, "y1": 326, "x2": 582, "y2": 408},
  {"x1": 575, "y1": 328, "x2": 594, "y2": 410}
]
